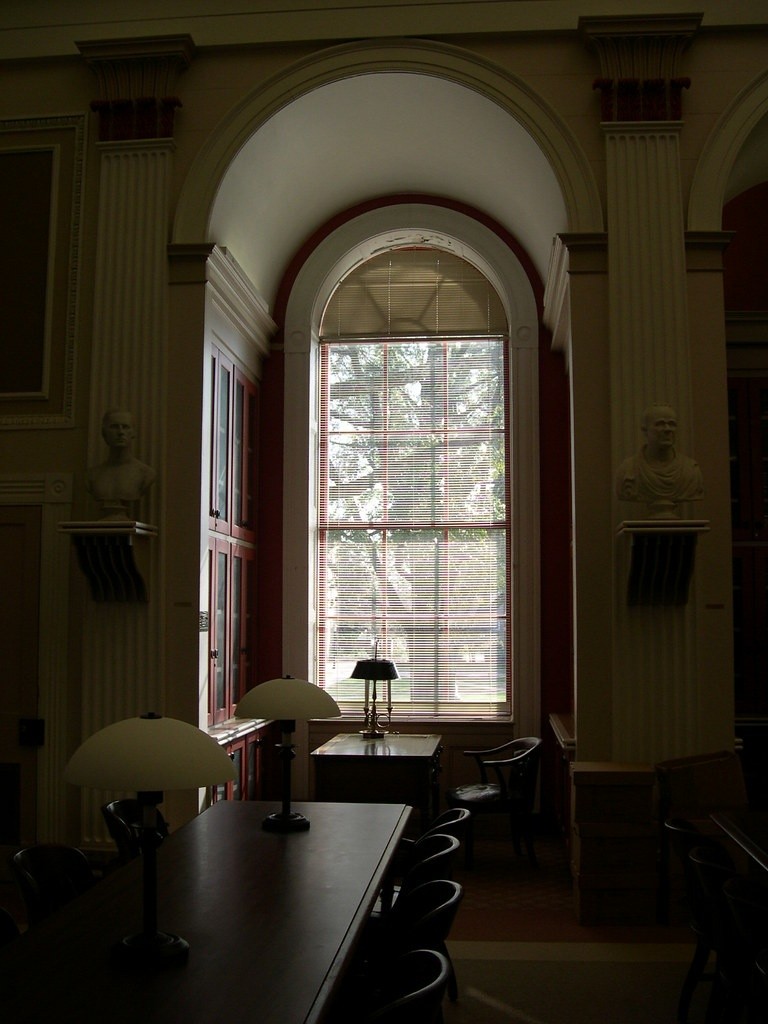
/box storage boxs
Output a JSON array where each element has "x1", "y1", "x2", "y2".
[{"x1": 551, "y1": 749, "x2": 748, "y2": 928}]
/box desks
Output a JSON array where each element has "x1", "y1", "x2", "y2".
[
  {"x1": 705, "y1": 804, "x2": 768, "y2": 876},
  {"x1": 309, "y1": 733, "x2": 443, "y2": 833},
  {"x1": 0, "y1": 800, "x2": 413, "y2": 1024},
  {"x1": 548, "y1": 710, "x2": 575, "y2": 818}
]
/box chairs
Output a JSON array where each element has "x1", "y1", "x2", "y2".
[
  {"x1": 336, "y1": 807, "x2": 471, "y2": 1024},
  {"x1": 0, "y1": 908, "x2": 20, "y2": 951},
  {"x1": 449, "y1": 736, "x2": 542, "y2": 870},
  {"x1": 660, "y1": 816, "x2": 767, "y2": 1024},
  {"x1": 101, "y1": 797, "x2": 171, "y2": 873},
  {"x1": 5, "y1": 840, "x2": 94, "y2": 931}
]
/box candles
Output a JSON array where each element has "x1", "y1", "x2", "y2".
[
  {"x1": 386, "y1": 680, "x2": 392, "y2": 708},
  {"x1": 365, "y1": 679, "x2": 369, "y2": 708}
]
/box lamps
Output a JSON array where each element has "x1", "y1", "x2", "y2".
[
  {"x1": 233, "y1": 674, "x2": 342, "y2": 835},
  {"x1": 66, "y1": 713, "x2": 234, "y2": 964},
  {"x1": 351, "y1": 660, "x2": 399, "y2": 738}
]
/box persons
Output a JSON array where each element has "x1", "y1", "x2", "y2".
[
  {"x1": 85, "y1": 407, "x2": 157, "y2": 511},
  {"x1": 615, "y1": 400, "x2": 705, "y2": 504}
]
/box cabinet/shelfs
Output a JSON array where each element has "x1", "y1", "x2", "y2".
[
  {"x1": 213, "y1": 723, "x2": 276, "y2": 802},
  {"x1": 208, "y1": 342, "x2": 262, "y2": 729}
]
